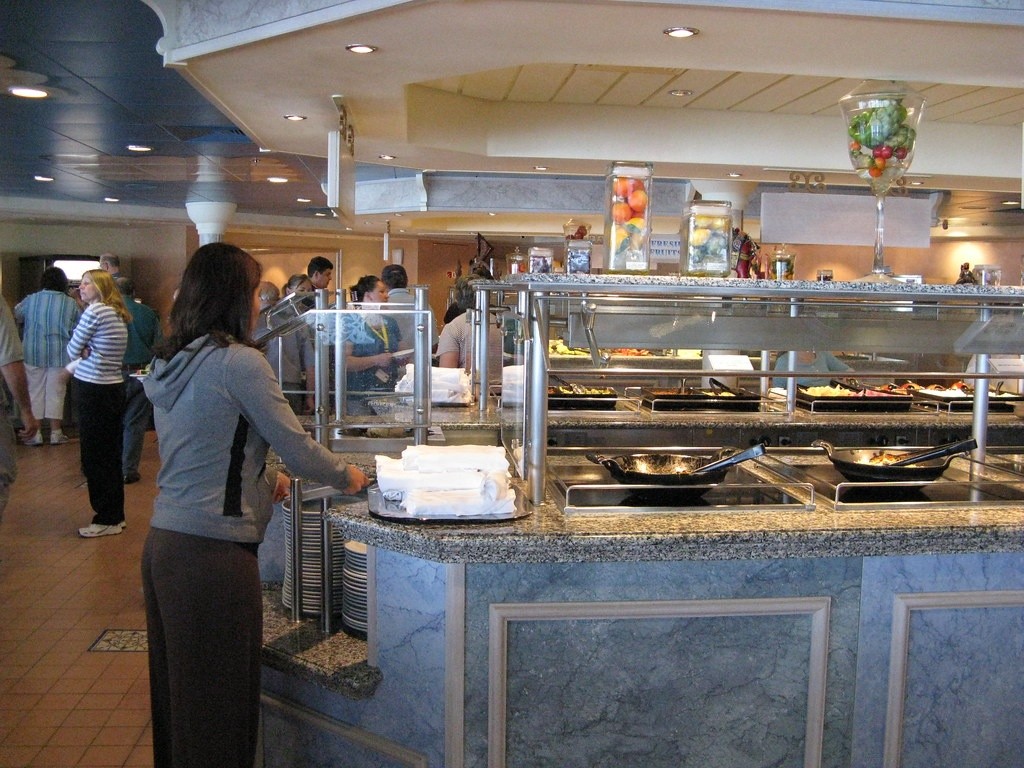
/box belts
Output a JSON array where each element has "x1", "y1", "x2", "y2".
[{"x1": 122, "y1": 363, "x2": 147, "y2": 372}]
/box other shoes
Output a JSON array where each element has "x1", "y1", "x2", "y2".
[{"x1": 124, "y1": 474, "x2": 140, "y2": 484}]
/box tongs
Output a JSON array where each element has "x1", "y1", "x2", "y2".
[
  {"x1": 680, "y1": 378, "x2": 686, "y2": 394},
  {"x1": 882, "y1": 438, "x2": 978, "y2": 466},
  {"x1": 709, "y1": 377, "x2": 745, "y2": 397},
  {"x1": 691, "y1": 443, "x2": 767, "y2": 474},
  {"x1": 549, "y1": 374, "x2": 573, "y2": 393},
  {"x1": 830, "y1": 380, "x2": 866, "y2": 395}
]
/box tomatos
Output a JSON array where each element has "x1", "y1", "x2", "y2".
[
  {"x1": 849, "y1": 141, "x2": 862, "y2": 151},
  {"x1": 869, "y1": 146, "x2": 907, "y2": 177}
]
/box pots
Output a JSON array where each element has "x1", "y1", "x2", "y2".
[
  {"x1": 811, "y1": 439, "x2": 970, "y2": 491},
  {"x1": 585, "y1": 447, "x2": 745, "y2": 501}
]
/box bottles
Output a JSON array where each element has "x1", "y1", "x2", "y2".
[
  {"x1": 679, "y1": 199, "x2": 733, "y2": 278},
  {"x1": 527, "y1": 247, "x2": 554, "y2": 274},
  {"x1": 602, "y1": 160, "x2": 654, "y2": 276},
  {"x1": 506, "y1": 246, "x2": 529, "y2": 275},
  {"x1": 564, "y1": 239, "x2": 593, "y2": 275},
  {"x1": 767, "y1": 243, "x2": 796, "y2": 281},
  {"x1": 562, "y1": 219, "x2": 592, "y2": 273}
]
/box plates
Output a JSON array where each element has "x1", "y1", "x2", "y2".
[{"x1": 280, "y1": 497, "x2": 368, "y2": 631}]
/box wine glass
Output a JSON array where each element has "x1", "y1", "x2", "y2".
[{"x1": 838, "y1": 79, "x2": 928, "y2": 284}]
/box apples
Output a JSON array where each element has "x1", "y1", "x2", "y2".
[{"x1": 611, "y1": 179, "x2": 648, "y2": 222}]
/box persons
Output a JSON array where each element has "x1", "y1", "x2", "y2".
[
  {"x1": 772, "y1": 350, "x2": 856, "y2": 390},
  {"x1": 960, "y1": 263, "x2": 975, "y2": 280},
  {"x1": 251, "y1": 255, "x2": 438, "y2": 417},
  {"x1": 14, "y1": 267, "x2": 80, "y2": 445},
  {"x1": 67, "y1": 253, "x2": 163, "y2": 484},
  {"x1": 435, "y1": 266, "x2": 517, "y2": 396},
  {"x1": 66, "y1": 269, "x2": 133, "y2": 537},
  {"x1": 141, "y1": 243, "x2": 371, "y2": 768},
  {"x1": 0, "y1": 293, "x2": 38, "y2": 515}
]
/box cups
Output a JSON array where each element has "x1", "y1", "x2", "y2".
[
  {"x1": 817, "y1": 269, "x2": 833, "y2": 282},
  {"x1": 974, "y1": 263, "x2": 1002, "y2": 287}
]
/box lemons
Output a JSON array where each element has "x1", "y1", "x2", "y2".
[
  {"x1": 605, "y1": 217, "x2": 647, "y2": 249},
  {"x1": 689, "y1": 215, "x2": 730, "y2": 246}
]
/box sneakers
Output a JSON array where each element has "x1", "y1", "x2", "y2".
[
  {"x1": 50, "y1": 433, "x2": 70, "y2": 444},
  {"x1": 78, "y1": 521, "x2": 127, "y2": 537},
  {"x1": 24, "y1": 434, "x2": 42, "y2": 445}
]
